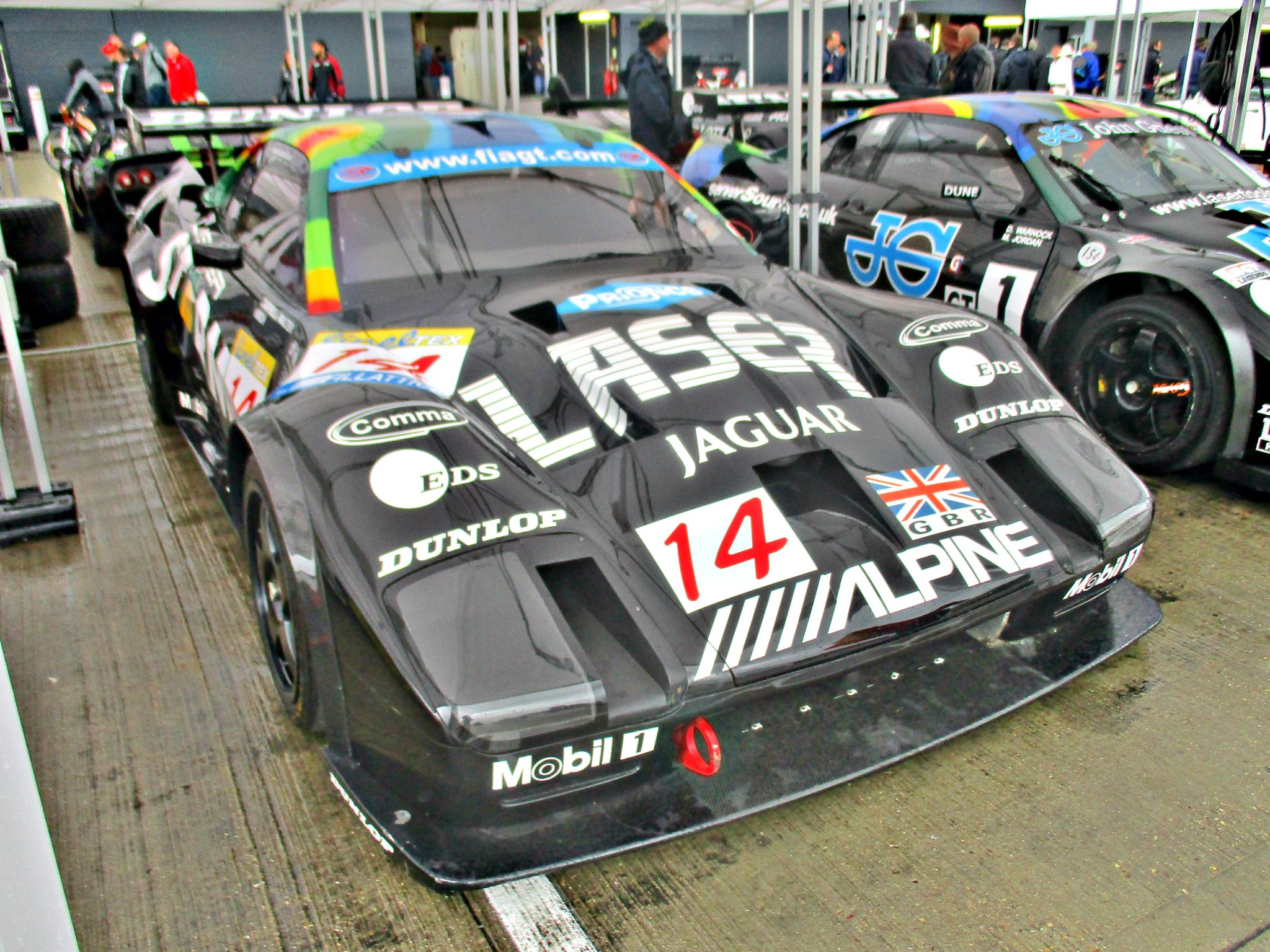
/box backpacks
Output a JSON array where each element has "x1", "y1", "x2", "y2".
[
  {"x1": 1198, "y1": 8, "x2": 1261, "y2": 107},
  {"x1": 1073, "y1": 55, "x2": 1094, "y2": 82}
]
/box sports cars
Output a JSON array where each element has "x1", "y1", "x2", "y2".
[
  {"x1": 58, "y1": 104, "x2": 249, "y2": 269},
  {"x1": 679, "y1": 81, "x2": 1269, "y2": 501},
  {"x1": 106, "y1": 105, "x2": 1162, "y2": 896}
]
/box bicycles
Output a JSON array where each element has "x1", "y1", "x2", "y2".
[{"x1": 42, "y1": 111, "x2": 98, "y2": 174}]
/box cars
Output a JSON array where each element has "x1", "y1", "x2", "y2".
[{"x1": 1153, "y1": 23, "x2": 1270, "y2": 163}]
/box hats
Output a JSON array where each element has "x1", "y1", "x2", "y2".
[
  {"x1": 1057, "y1": 46, "x2": 1076, "y2": 57},
  {"x1": 132, "y1": 33, "x2": 146, "y2": 47},
  {"x1": 638, "y1": 20, "x2": 668, "y2": 45},
  {"x1": 898, "y1": 12, "x2": 917, "y2": 27},
  {"x1": 1196, "y1": 36, "x2": 1211, "y2": 47},
  {"x1": 102, "y1": 36, "x2": 122, "y2": 55}
]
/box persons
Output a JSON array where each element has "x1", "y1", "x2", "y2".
[
  {"x1": 1176, "y1": 37, "x2": 1208, "y2": 99},
  {"x1": 1140, "y1": 38, "x2": 1163, "y2": 105},
  {"x1": 617, "y1": 17, "x2": 677, "y2": 165},
  {"x1": 803, "y1": 12, "x2": 1099, "y2": 100},
  {"x1": 541, "y1": 76, "x2": 578, "y2": 117},
  {"x1": 102, "y1": 32, "x2": 198, "y2": 113},
  {"x1": 58, "y1": 58, "x2": 114, "y2": 155},
  {"x1": 271, "y1": 39, "x2": 346, "y2": 104},
  {"x1": 527, "y1": 36, "x2": 545, "y2": 94},
  {"x1": 416, "y1": 43, "x2": 451, "y2": 98}
]
